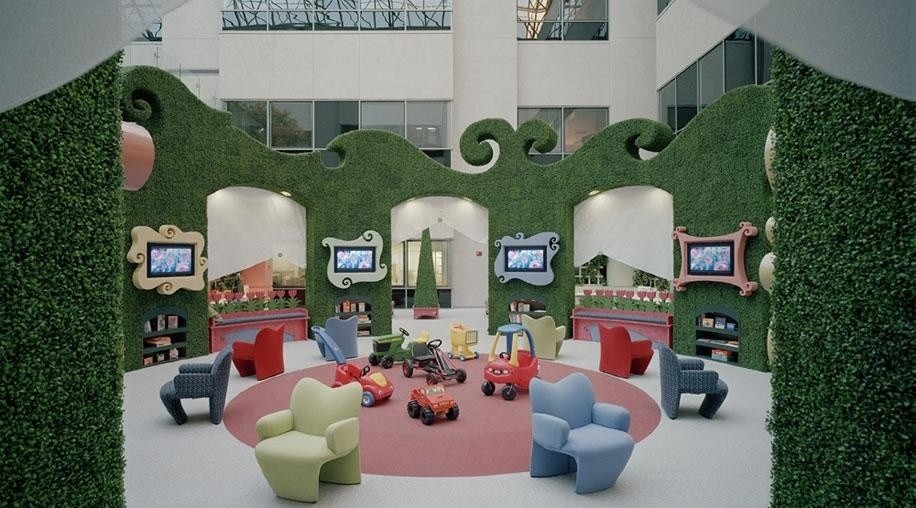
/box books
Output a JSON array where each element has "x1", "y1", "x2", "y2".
[
  {"x1": 336, "y1": 300, "x2": 371, "y2": 336},
  {"x1": 143, "y1": 314, "x2": 179, "y2": 366},
  {"x1": 509, "y1": 301, "x2": 530, "y2": 323},
  {"x1": 698, "y1": 313, "x2": 739, "y2": 362}
]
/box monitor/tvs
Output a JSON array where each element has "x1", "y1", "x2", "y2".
[
  {"x1": 687, "y1": 242, "x2": 734, "y2": 276},
  {"x1": 147, "y1": 242, "x2": 194, "y2": 277},
  {"x1": 505, "y1": 246, "x2": 547, "y2": 272},
  {"x1": 334, "y1": 247, "x2": 375, "y2": 272}
]
